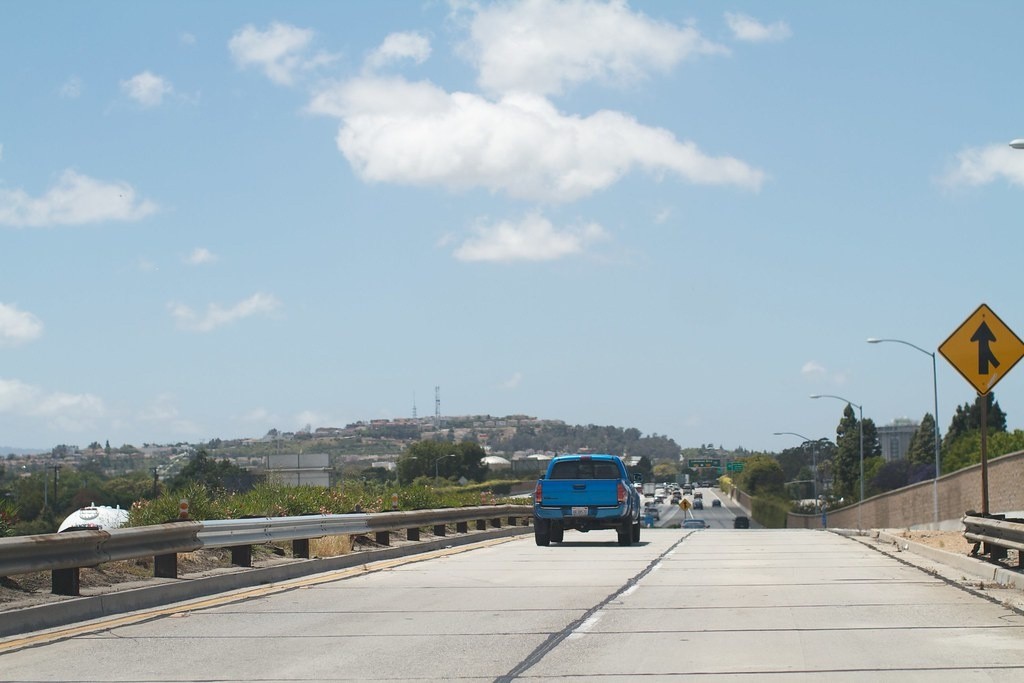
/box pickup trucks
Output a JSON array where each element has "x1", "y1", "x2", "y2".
[{"x1": 679, "y1": 519, "x2": 710, "y2": 529}]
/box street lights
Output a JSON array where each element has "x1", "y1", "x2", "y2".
[
  {"x1": 148, "y1": 467, "x2": 162, "y2": 498},
  {"x1": 867, "y1": 339, "x2": 941, "y2": 477},
  {"x1": 49, "y1": 464, "x2": 63, "y2": 506},
  {"x1": 774, "y1": 432, "x2": 818, "y2": 514},
  {"x1": 810, "y1": 394, "x2": 864, "y2": 501},
  {"x1": 436, "y1": 455, "x2": 455, "y2": 477}
]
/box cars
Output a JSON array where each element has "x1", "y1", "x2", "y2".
[
  {"x1": 702, "y1": 482, "x2": 709, "y2": 488},
  {"x1": 671, "y1": 498, "x2": 679, "y2": 505},
  {"x1": 646, "y1": 507, "x2": 661, "y2": 520},
  {"x1": 639, "y1": 514, "x2": 654, "y2": 528},
  {"x1": 693, "y1": 500, "x2": 703, "y2": 510},
  {"x1": 633, "y1": 483, "x2": 642, "y2": 487},
  {"x1": 654, "y1": 497, "x2": 663, "y2": 504},
  {"x1": 694, "y1": 492, "x2": 702, "y2": 499},
  {"x1": 654, "y1": 483, "x2": 682, "y2": 500},
  {"x1": 733, "y1": 516, "x2": 750, "y2": 529},
  {"x1": 712, "y1": 500, "x2": 721, "y2": 507}
]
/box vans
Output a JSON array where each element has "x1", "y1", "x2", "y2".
[{"x1": 683, "y1": 484, "x2": 692, "y2": 495}]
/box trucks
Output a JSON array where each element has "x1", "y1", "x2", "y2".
[
  {"x1": 643, "y1": 483, "x2": 655, "y2": 497},
  {"x1": 532, "y1": 454, "x2": 641, "y2": 547}
]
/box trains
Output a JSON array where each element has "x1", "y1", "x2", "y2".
[{"x1": 57, "y1": 502, "x2": 131, "y2": 532}]
